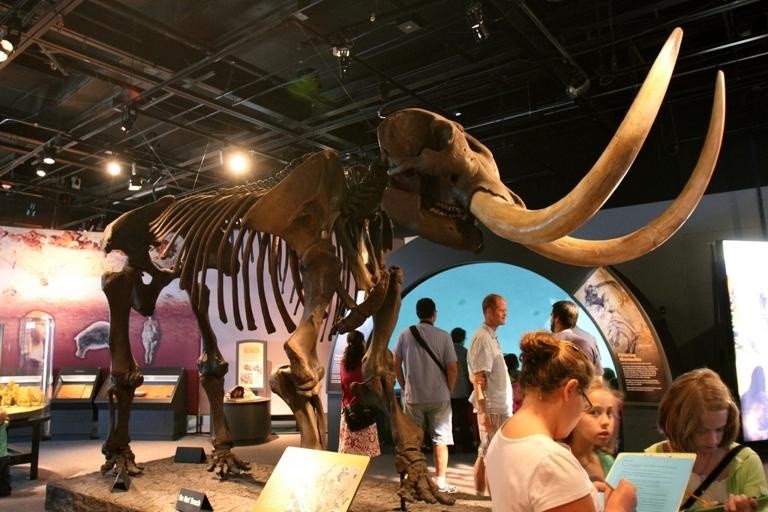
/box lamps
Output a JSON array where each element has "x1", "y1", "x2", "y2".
[
  {"x1": 127, "y1": 164, "x2": 167, "y2": 189},
  {"x1": 465, "y1": 5, "x2": 489, "y2": 47},
  {"x1": 0, "y1": 13, "x2": 24, "y2": 63},
  {"x1": 37, "y1": 144, "x2": 58, "y2": 179},
  {"x1": 331, "y1": 37, "x2": 353, "y2": 80}
]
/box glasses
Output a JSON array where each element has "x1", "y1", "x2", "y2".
[{"x1": 579, "y1": 387, "x2": 593, "y2": 412}]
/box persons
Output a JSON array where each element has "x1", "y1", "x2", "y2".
[
  {"x1": 338, "y1": 295, "x2": 527, "y2": 492},
  {"x1": 644, "y1": 367, "x2": 767, "y2": 512},
  {"x1": 483, "y1": 301, "x2": 637, "y2": 512}
]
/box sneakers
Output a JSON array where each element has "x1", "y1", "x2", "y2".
[{"x1": 438, "y1": 484, "x2": 457, "y2": 493}]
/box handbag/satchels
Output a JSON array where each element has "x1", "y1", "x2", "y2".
[{"x1": 344, "y1": 401, "x2": 377, "y2": 433}]
